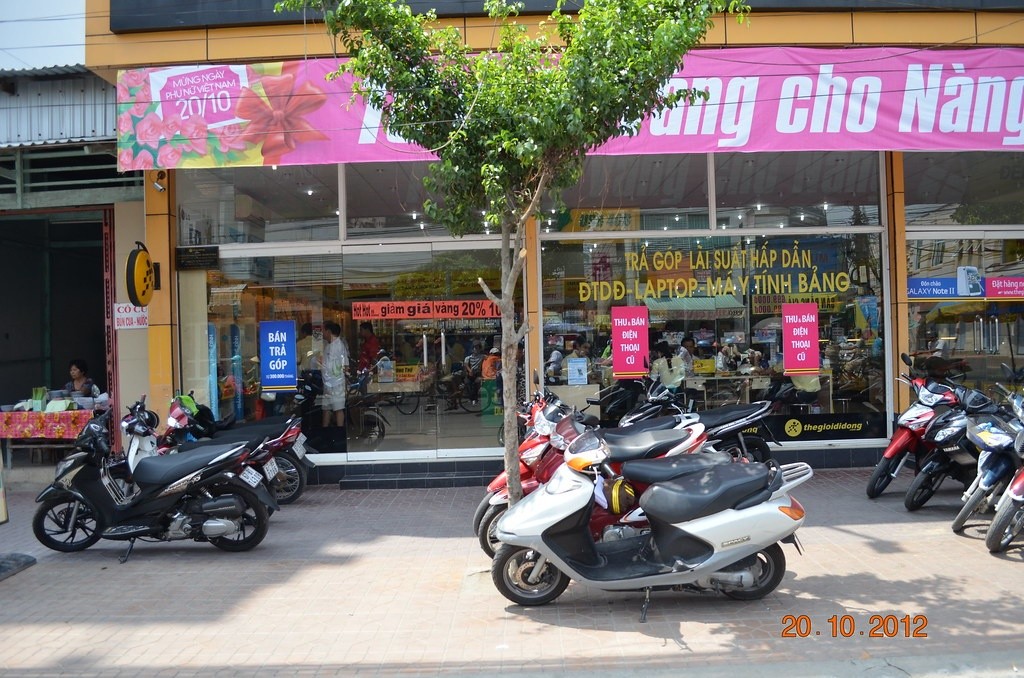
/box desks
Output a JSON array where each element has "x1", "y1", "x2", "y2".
[
  {"x1": 0, "y1": 412, "x2": 95, "y2": 469},
  {"x1": 684, "y1": 376, "x2": 768, "y2": 412}
]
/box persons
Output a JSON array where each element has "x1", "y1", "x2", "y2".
[
  {"x1": 365, "y1": 348, "x2": 393, "y2": 394},
  {"x1": 912, "y1": 330, "x2": 951, "y2": 378},
  {"x1": 61, "y1": 359, "x2": 94, "y2": 397},
  {"x1": 839, "y1": 329, "x2": 883, "y2": 390},
  {"x1": 721, "y1": 328, "x2": 822, "y2": 412},
  {"x1": 561, "y1": 327, "x2": 704, "y2": 414},
  {"x1": 296, "y1": 322, "x2": 381, "y2": 427},
  {"x1": 668, "y1": 335, "x2": 749, "y2": 372},
  {"x1": 416, "y1": 337, "x2": 563, "y2": 411}
]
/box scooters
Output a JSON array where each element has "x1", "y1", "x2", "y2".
[
  {"x1": 491, "y1": 396, "x2": 815, "y2": 606},
  {"x1": 615, "y1": 375, "x2": 782, "y2": 465},
  {"x1": 467, "y1": 368, "x2": 756, "y2": 564}
]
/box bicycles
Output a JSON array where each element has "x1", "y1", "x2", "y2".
[{"x1": 342, "y1": 349, "x2": 495, "y2": 418}]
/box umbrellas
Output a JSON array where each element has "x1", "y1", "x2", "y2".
[{"x1": 925, "y1": 274, "x2": 1024, "y2": 375}]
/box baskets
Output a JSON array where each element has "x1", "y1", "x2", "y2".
[{"x1": 965, "y1": 411, "x2": 1018, "y2": 452}]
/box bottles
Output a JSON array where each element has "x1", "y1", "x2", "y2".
[
  {"x1": 727, "y1": 343, "x2": 737, "y2": 370},
  {"x1": 760, "y1": 359, "x2": 769, "y2": 369}
]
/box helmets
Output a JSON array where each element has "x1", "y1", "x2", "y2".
[{"x1": 144, "y1": 410, "x2": 159, "y2": 429}]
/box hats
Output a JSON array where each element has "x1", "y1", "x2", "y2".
[
  {"x1": 854, "y1": 327, "x2": 861, "y2": 333},
  {"x1": 472, "y1": 340, "x2": 481, "y2": 346},
  {"x1": 433, "y1": 338, "x2": 441, "y2": 345},
  {"x1": 490, "y1": 348, "x2": 500, "y2": 354},
  {"x1": 444, "y1": 318, "x2": 459, "y2": 320},
  {"x1": 377, "y1": 349, "x2": 386, "y2": 354},
  {"x1": 415, "y1": 339, "x2": 423, "y2": 347}
]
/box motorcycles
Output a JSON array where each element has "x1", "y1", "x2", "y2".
[
  {"x1": 155, "y1": 388, "x2": 317, "y2": 509},
  {"x1": 497, "y1": 386, "x2": 560, "y2": 448},
  {"x1": 111, "y1": 393, "x2": 278, "y2": 528},
  {"x1": 865, "y1": 351, "x2": 1024, "y2": 552},
  {"x1": 260, "y1": 374, "x2": 393, "y2": 455},
  {"x1": 32, "y1": 383, "x2": 279, "y2": 565}
]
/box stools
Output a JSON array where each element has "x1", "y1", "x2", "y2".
[
  {"x1": 792, "y1": 404, "x2": 813, "y2": 417},
  {"x1": 833, "y1": 398, "x2": 852, "y2": 413}
]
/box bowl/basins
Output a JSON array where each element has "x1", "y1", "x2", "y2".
[
  {"x1": 1, "y1": 399, "x2": 33, "y2": 411},
  {"x1": 49, "y1": 390, "x2": 93, "y2": 410}
]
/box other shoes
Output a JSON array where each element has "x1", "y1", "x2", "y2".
[
  {"x1": 444, "y1": 405, "x2": 458, "y2": 410},
  {"x1": 449, "y1": 391, "x2": 462, "y2": 398}
]
